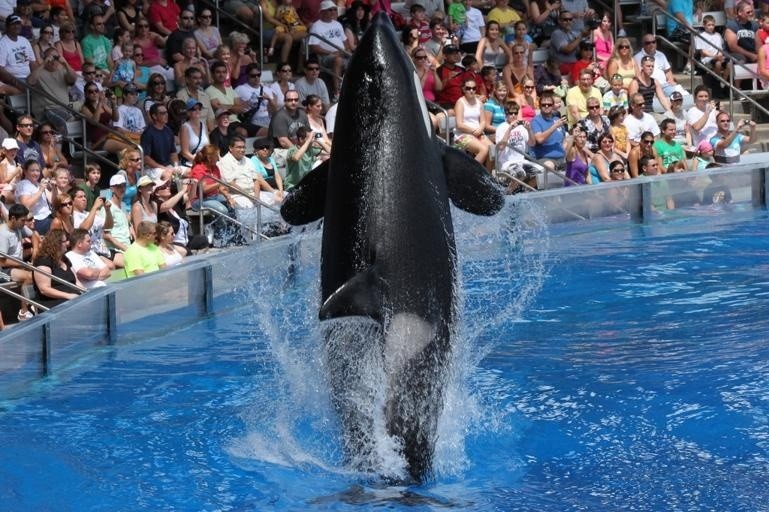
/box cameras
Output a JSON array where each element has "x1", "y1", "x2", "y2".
[
  {"x1": 447, "y1": 35, "x2": 453, "y2": 39},
  {"x1": 100, "y1": 197, "x2": 106, "y2": 203},
  {"x1": 53, "y1": 55, "x2": 59, "y2": 60},
  {"x1": 54, "y1": 135, "x2": 63, "y2": 142},
  {"x1": 44, "y1": 180, "x2": 51, "y2": 184},
  {"x1": 518, "y1": 120, "x2": 525, "y2": 125},
  {"x1": 315, "y1": 133, "x2": 322, "y2": 138},
  {"x1": 105, "y1": 88, "x2": 113, "y2": 98},
  {"x1": 188, "y1": 179, "x2": 194, "y2": 184},
  {"x1": 744, "y1": 119, "x2": 750, "y2": 125}
]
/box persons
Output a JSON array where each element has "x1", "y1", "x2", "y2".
[
  {"x1": 403, "y1": 0, "x2": 757, "y2": 213},
  {"x1": 2, "y1": 0, "x2": 394, "y2": 326},
  {"x1": 619, "y1": 0, "x2": 769, "y2": 123}
]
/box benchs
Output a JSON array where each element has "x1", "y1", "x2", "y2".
[{"x1": 0, "y1": 0, "x2": 769, "y2": 313}]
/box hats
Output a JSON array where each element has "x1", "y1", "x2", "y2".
[
  {"x1": 319, "y1": 1, "x2": 337, "y2": 15},
  {"x1": 215, "y1": 108, "x2": 231, "y2": 118},
  {"x1": 110, "y1": 173, "x2": 169, "y2": 192},
  {"x1": 443, "y1": 44, "x2": 460, "y2": 54},
  {"x1": 1, "y1": 138, "x2": 20, "y2": 150},
  {"x1": 5, "y1": 15, "x2": 22, "y2": 25}
]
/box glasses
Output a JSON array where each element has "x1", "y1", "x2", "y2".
[
  {"x1": 746, "y1": 10, "x2": 754, "y2": 13},
  {"x1": 42, "y1": 15, "x2": 212, "y2": 35},
  {"x1": 579, "y1": 40, "x2": 656, "y2": 51},
  {"x1": 328, "y1": 9, "x2": 337, "y2": 11},
  {"x1": 61, "y1": 201, "x2": 72, "y2": 206},
  {"x1": 42, "y1": 130, "x2": 52, "y2": 134},
  {"x1": 508, "y1": 85, "x2": 553, "y2": 114},
  {"x1": 250, "y1": 66, "x2": 323, "y2": 108},
  {"x1": 86, "y1": 71, "x2": 202, "y2": 115},
  {"x1": 135, "y1": 53, "x2": 144, "y2": 57},
  {"x1": 20, "y1": 124, "x2": 33, "y2": 127},
  {"x1": 157, "y1": 183, "x2": 169, "y2": 190},
  {"x1": 588, "y1": 94, "x2": 730, "y2": 174},
  {"x1": 131, "y1": 158, "x2": 141, "y2": 162},
  {"x1": 564, "y1": 17, "x2": 573, "y2": 22},
  {"x1": 417, "y1": 56, "x2": 427, "y2": 60},
  {"x1": 259, "y1": 145, "x2": 270, "y2": 150},
  {"x1": 514, "y1": 51, "x2": 524, "y2": 55},
  {"x1": 465, "y1": 86, "x2": 477, "y2": 90}
]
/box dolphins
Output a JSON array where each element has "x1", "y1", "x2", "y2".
[{"x1": 277, "y1": 9, "x2": 505, "y2": 509}]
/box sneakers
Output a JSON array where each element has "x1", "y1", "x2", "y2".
[
  {"x1": 734, "y1": 88, "x2": 746, "y2": 101},
  {"x1": 682, "y1": 64, "x2": 699, "y2": 75}
]
